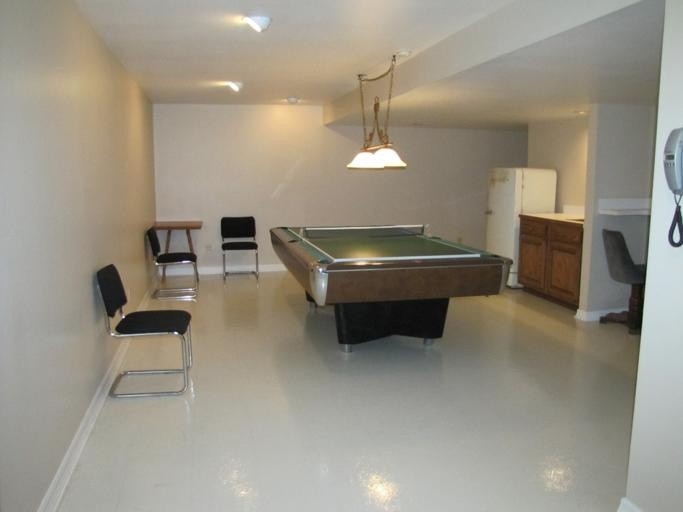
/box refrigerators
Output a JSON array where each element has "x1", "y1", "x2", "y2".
[{"x1": 486, "y1": 167, "x2": 557, "y2": 287}]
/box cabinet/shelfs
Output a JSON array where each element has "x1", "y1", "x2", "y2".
[{"x1": 516, "y1": 211, "x2": 583, "y2": 310}]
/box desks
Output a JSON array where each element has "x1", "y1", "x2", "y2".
[
  {"x1": 151, "y1": 220, "x2": 202, "y2": 285},
  {"x1": 267, "y1": 222, "x2": 515, "y2": 353}
]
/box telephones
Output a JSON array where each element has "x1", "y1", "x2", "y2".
[{"x1": 664, "y1": 128, "x2": 683, "y2": 196}]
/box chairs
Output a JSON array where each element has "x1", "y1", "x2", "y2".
[
  {"x1": 218, "y1": 216, "x2": 259, "y2": 282},
  {"x1": 96, "y1": 264, "x2": 192, "y2": 398},
  {"x1": 598, "y1": 228, "x2": 647, "y2": 334},
  {"x1": 145, "y1": 229, "x2": 199, "y2": 302}
]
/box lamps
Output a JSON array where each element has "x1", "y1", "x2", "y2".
[{"x1": 345, "y1": 57, "x2": 407, "y2": 172}]
